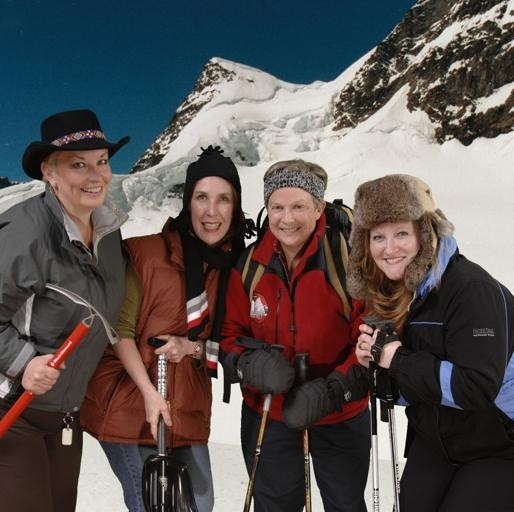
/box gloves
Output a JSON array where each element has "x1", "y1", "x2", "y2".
[
  {"x1": 282, "y1": 378, "x2": 336, "y2": 428},
  {"x1": 238, "y1": 348, "x2": 295, "y2": 394}
]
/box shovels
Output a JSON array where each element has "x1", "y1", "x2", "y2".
[{"x1": 141, "y1": 337, "x2": 199, "y2": 512}]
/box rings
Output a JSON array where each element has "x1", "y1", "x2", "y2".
[
  {"x1": 358, "y1": 342, "x2": 364, "y2": 350},
  {"x1": 165, "y1": 352, "x2": 167, "y2": 357}
]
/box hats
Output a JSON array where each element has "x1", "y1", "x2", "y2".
[
  {"x1": 345, "y1": 174, "x2": 453, "y2": 299},
  {"x1": 183, "y1": 145, "x2": 241, "y2": 208},
  {"x1": 23, "y1": 110, "x2": 130, "y2": 180}
]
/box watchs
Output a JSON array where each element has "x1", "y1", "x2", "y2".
[{"x1": 192, "y1": 341, "x2": 200, "y2": 357}]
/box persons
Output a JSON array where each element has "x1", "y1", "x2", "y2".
[
  {"x1": 80, "y1": 145, "x2": 245, "y2": 512},
  {"x1": 345, "y1": 173, "x2": 514, "y2": 512},
  {"x1": 0, "y1": 108, "x2": 131, "y2": 512},
  {"x1": 218, "y1": 158, "x2": 375, "y2": 512}
]
